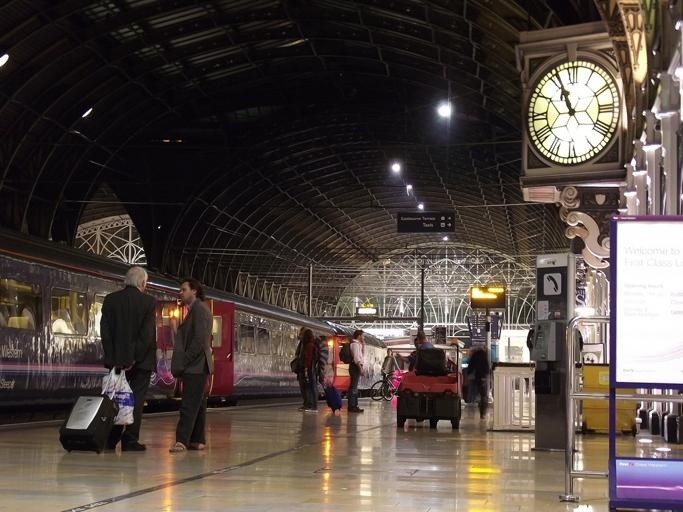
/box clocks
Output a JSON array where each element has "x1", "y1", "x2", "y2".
[{"x1": 513, "y1": 20, "x2": 626, "y2": 187}]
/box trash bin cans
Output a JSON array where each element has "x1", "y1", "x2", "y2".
[{"x1": 582, "y1": 364, "x2": 637, "y2": 437}]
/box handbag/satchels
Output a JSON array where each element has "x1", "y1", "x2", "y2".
[
  {"x1": 291, "y1": 359, "x2": 303, "y2": 373},
  {"x1": 101, "y1": 367, "x2": 134, "y2": 424}
]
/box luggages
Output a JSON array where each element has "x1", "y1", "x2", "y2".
[
  {"x1": 60, "y1": 367, "x2": 124, "y2": 453},
  {"x1": 322, "y1": 382, "x2": 341, "y2": 412}
]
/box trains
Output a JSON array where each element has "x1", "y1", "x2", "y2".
[{"x1": 1, "y1": 226, "x2": 388, "y2": 406}]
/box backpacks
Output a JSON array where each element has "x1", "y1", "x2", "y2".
[{"x1": 339, "y1": 342, "x2": 360, "y2": 364}]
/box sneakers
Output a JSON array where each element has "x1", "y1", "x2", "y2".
[
  {"x1": 189, "y1": 442, "x2": 204, "y2": 450},
  {"x1": 348, "y1": 407, "x2": 364, "y2": 412},
  {"x1": 299, "y1": 406, "x2": 318, "y2": 412},
  {"x1": 170, "y1": 442, "x2": 185, "y2": 451}
]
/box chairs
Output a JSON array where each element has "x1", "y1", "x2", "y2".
[{"x1": 7, "y1": 316, "x2": 29, "y2": 328}]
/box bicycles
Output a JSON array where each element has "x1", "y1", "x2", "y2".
[{"x1": 370, "y1": 368, "x2": 409, "y2": 401}]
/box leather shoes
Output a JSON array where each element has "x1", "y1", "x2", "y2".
[
  {"x1": 122, "y1": 442, "x2": 145, "y2": 451},
  {"x1": 106, "y1": 440, "x2": 117, "y2": 449}
]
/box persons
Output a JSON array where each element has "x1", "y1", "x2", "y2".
[
  {"x1": 167, "y1": 279, "x2": 213, "y2": 452},
  {"x1": 101, "y1": 266, "x2": 157, "y2": 451},
  {"x1": 396, "y1": 330, "x2": 491, "y2": 420},
  {"x1": 381, "y1": 348, "x2": 400, "y2": 400},
  {"x1": 347, "y1": 330, "x2": 371, "y2": 413},
  {"x1": 304, "y1": 330, "x2": 320, "y2": 412},
  {"x1": 293, "y1": 326, "x2": 313, "y2": 409}
]
